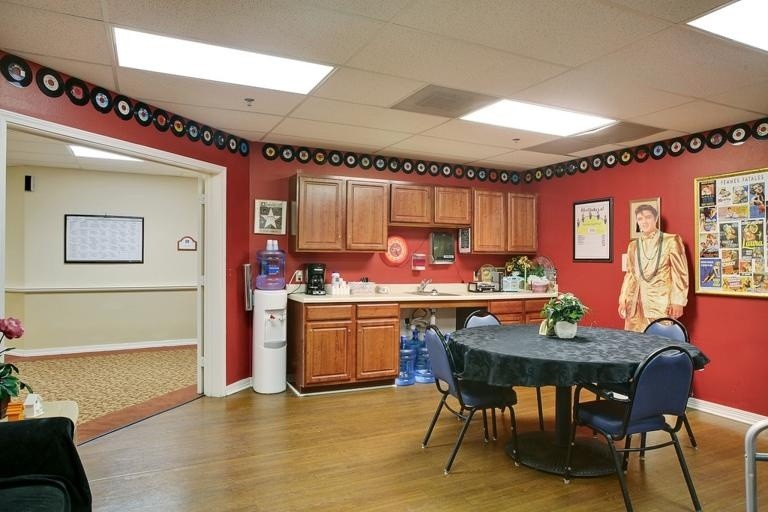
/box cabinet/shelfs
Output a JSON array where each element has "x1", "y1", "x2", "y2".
[
  {"x1": 289, "y1": 172, "x2": 388, "y2": 253},
  {"x1": 472, "y1": 187, "x2": 537, "y2": 254},
  {"x1": 389, "y1": 179, "x2": 473, "y2": 228},
  {"x1": 488, "y1": 300, "x2": 549, "y2": 324},
  {"x1": 287, "y1": 296, "x2": 400, "y2": 393}
]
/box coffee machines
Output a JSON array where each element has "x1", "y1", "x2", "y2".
[{"x1": 305, "y1": 263, "x2": 325, "y2": 294}]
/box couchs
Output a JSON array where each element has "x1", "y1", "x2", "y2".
[{"x1": 0, "y1": 416, "x2": 93, "y2": 512}]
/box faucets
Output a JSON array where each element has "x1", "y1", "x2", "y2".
[{"x1": 417, "y1": 278, "x2": 432, "y2": 292}]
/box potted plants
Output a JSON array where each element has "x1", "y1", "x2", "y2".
[{"x1": 541, "y1": 290, "x2": 588, "y2": 341}]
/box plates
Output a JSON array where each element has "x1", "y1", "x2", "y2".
[{"x1": 379, "y1": 233, "x2": 410, "y2": 268}]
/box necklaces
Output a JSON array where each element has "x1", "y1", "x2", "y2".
[{"x1": 637, "y1": 231, "x2": 663, "y2": 283}]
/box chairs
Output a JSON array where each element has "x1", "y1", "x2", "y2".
[
  {"x1": 463, "y1": 310, "x2": 502, "y2": 441},
  {"x1": 422, "y1": 324, "x2": 519, "y2": 475},
  {"x1": 563, "y1": 345, "x2": 705, "y2": 511},
  {"x1": 593, "y1": 317, "x2": 698, "y2": 449}
]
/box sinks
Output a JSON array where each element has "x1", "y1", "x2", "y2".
[{"x1": 405, "y1": 291, "x2": 458, "y2": 296}]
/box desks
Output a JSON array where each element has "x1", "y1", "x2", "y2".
[
  {"x1": 18, "y1": 399, "x2": 78, "y2": 424},
  {"x1": 446, "y1": 325, "x2": 708, "y2": 475}
]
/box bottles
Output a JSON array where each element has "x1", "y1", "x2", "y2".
[{"x1": 340, "y1": 281, "x2": 347, "y2": 288}]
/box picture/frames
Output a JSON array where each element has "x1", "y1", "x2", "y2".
[
  {"x1": 253, "y1": 198, "x2": 287, "y2": 236},
  {"x1": 627, "y1": 197, "x2": 660, "y2": 242},
  {"x1": 693, "y1": 168, "x2": 768, "y2": 298},
  {"x1": 573, "y1": 198, "x2": 613, "y2": 263}
]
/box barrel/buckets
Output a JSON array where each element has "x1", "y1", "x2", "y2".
[
  {"x1": 408, "y1": 330, "x2": 424, "y2": 349},
  {"x1": 416, "y1": 335, "x2": 436, "y2": 383},
  {"x1": 255, "y1": 250, "x2": 286, "y2": 290},
  {"x1": 395, "y1": 335, "x2": 416, "y2": 386}
]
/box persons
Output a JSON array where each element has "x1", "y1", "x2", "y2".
[
  {"x1": 618, "y1": 204, "x2": 689, "y2": 333},
  {"x1": 726, "y1": 207, "x2": 737, "y2": 218},
  {"x1": 701, "y1": 234, "x2": 716, "y2": 255},
  {"x1": 703, "y1": 262, "x2": 720, "y2": 283},
  {"x1": 751, "y1": 183, "x2": 764, "y2": 206},
  {"x1": 704, "y1": 207, "x2": 716, "y2": 231}
]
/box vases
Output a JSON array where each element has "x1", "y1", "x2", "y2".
[
  {"x1": 510, "y1": 271, "x2": 521, "y2": 276},
  {"x1": 0, "y1": 393, "x2": 11, "y2": 418}
]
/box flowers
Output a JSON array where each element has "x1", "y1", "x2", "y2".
[
  {"x1": 504, "y1": 255, "x2": 532, "y2": 274},
  {"x1": 0, "y1": 317, "x2": 33, "y2": 402}
]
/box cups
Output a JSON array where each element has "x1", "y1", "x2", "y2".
[{"x1": 332, "y1": 272, "x2": 339, "y2": 284}]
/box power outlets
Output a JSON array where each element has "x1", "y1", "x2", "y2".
[{"x1": 296, "y1": 270, "x2": 303, "y2": 282}]
